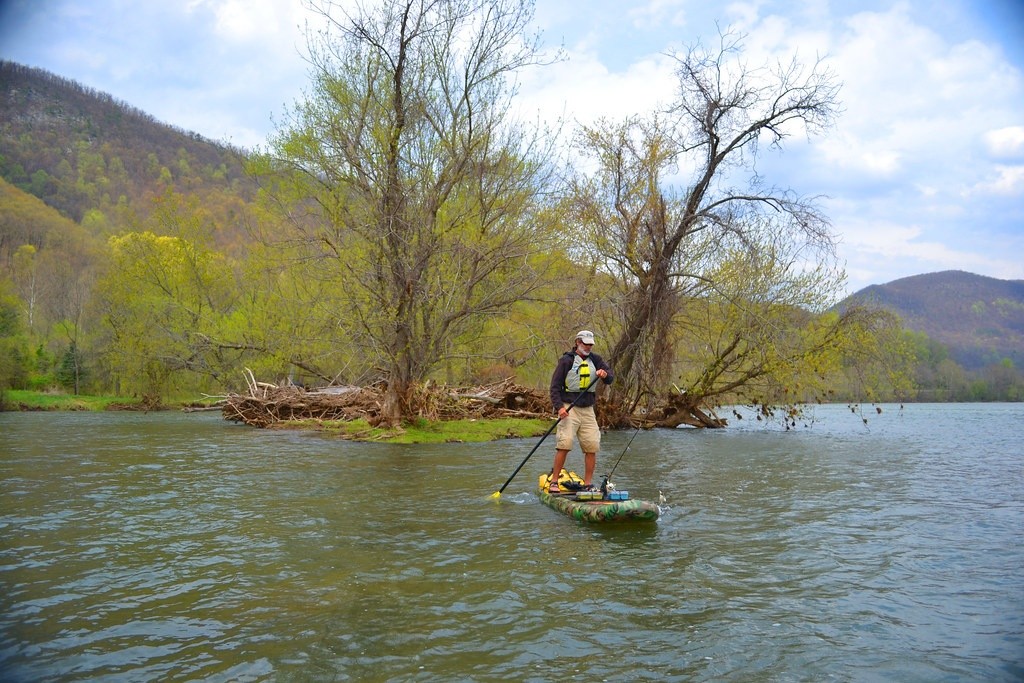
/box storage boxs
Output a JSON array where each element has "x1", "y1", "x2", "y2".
[
  {"x1": 576, "y1": 491, "x2": 602, "y2": 499},
  {"x1": 608, "y1": 490, "x2": 629, "y2": 499}
]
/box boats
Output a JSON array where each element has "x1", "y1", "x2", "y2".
[{"x1": 538, "y1": 468, "x2": 660, "y2": 524}]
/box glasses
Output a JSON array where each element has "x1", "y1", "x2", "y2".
[{"x1": 582, "y1": 341, "x2": 593, "y2": 346}]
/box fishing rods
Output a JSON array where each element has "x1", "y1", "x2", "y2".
[{"x1": 596, "y1": 375, "x2": 682, "y2": 492}]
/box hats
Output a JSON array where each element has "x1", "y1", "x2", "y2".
[{"x1": 575, "y1": 331, "x2": 595, "y2": 344}]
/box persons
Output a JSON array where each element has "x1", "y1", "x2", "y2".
[
  {"x1": 305, "y1": 383, "x2": 315, "y2": 393},
  {"x1": 548, "y1": 330, "x2": 614, "y2": 493}
]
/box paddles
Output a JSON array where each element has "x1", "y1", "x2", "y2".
[{"x1": 488, "y1": 374, "x2": 601, "y2": 501}]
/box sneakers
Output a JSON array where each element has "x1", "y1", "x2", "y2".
[
  {"x1": 548, "y1": 482, "x2": 560, "y2": 493},
  {"x1": 585, "y1": 484, "x2": 597, "y2": 492}
]
